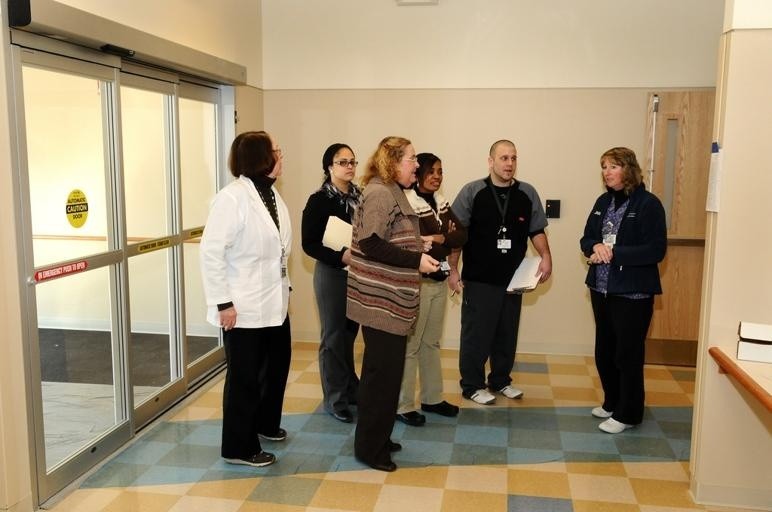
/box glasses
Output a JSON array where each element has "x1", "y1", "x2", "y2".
[
  {"x1": 332, "y1": 159, "x2": 359, "y2": 168},
  {"x1": 398, "y1": 156, "x2": 417, "y2": 164},
  {"x1": 270, "y1": 147, "x2": 283, "y2": 156}
]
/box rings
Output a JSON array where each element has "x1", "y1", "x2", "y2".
[{"x1": 426, "y1": 272, "x2": 430, "y2": 275}]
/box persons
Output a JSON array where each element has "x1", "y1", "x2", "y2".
[
  {"x1": 345, "y1": 137, "x2": 441, "y2": 472},
  {"x1": 447, "y1": 140, "x2": 552, "y2": 405},
  {"x1": 198, "y1": 131, "x2": 294, "y2": 467},
  {"x1": 396, "y1": 154, "x2": 468, "y2": 427},
  {"x1": 301, "y1": 144, "x2": 363, "y2": 423},
  {"x1": 580, "y1": 148, "x2": 666, "y2": 434}
]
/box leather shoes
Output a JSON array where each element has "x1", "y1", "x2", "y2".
[
  {"x1": 330, "y1": 406, "x2": 356, "y2": 426},
  {"x1": 365, "y1": 459, "x2": 398, "y2": 473},
  {"x1": 388, "y1": 439, "x2": 403, "y2": 454}
]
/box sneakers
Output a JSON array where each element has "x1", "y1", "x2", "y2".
[
  {"x1": 589, "y1": 404, "x2": 617, "y2": 419},
  {"x1": 596, "y1": 417, "x2": 640, "y2": 436},
  {"x1": 396, "y1": 408, "x2": 429, "y2": 428},
  {"x1": 258, "y1": 424, "x2": 287, "y2": 444},
  {"x1": 221, "y1": 450, "x2": 276, "y2": 469},
  {"x1": 420, "y1": 398, "x2": 460, "y2": 419},
  {"x1": 496, "y1": 382, "x2": 526, "y2": 400},
  {"x1": 467, "y1": 386, "x2": 497, "y2": 407}
]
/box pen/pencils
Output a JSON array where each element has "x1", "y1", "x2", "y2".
[{"x1": 451, "y1": 280, "x2": 460, "y2": 297}]
